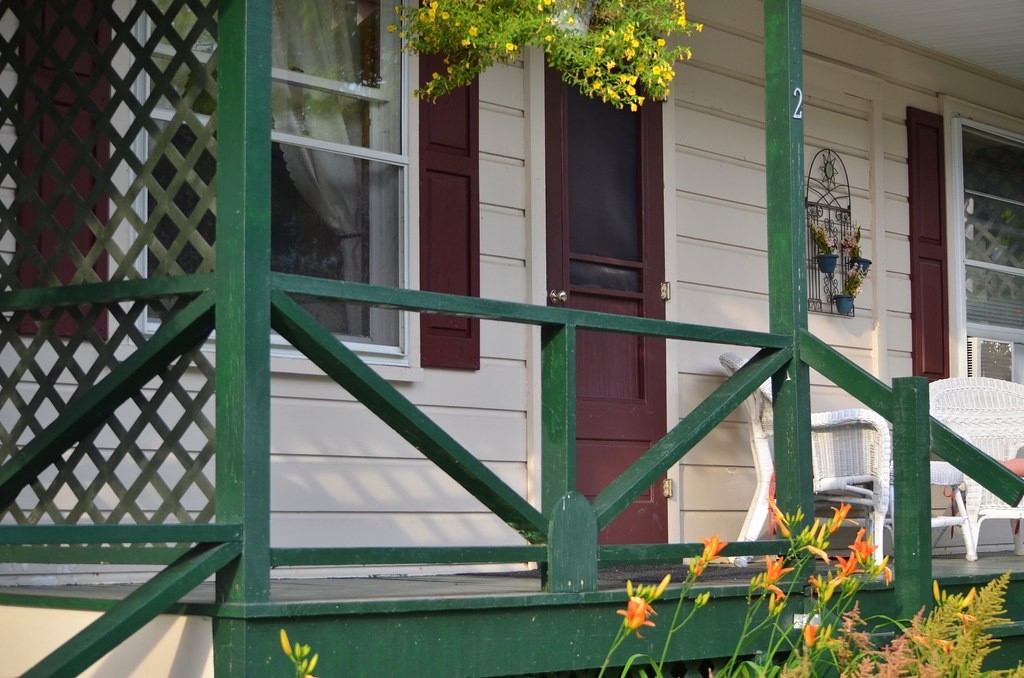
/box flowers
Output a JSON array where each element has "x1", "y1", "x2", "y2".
[
  {"x1": 840, "y1": 227, "x2": 864, "y2": 257},
  {"x1": 807, "y1": 223, "x2": 839, "y2": 255},
  {"x1": 839, "y1": 262, "x2": 872, "y2": 295},
  {"x1": 385, "y1": 0, "x2": 705, "y2": 112}
]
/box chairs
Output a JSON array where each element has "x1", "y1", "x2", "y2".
[
  {"x1": 928, "y1": 376, "x2": 1024, "y2": 557},
  {"x1": 718, "y1": 351, "x2": 892, "y2": 571},
  {"x1": 885, "y1": 458, "x2": 977, "y2": 561}
]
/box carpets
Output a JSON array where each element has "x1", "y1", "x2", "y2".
[{"x1": 599, "y1": 566, "x2": 766, "y2": 582}]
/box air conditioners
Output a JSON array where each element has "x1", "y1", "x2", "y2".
[{"x1": 967, "y1": 338, "x2": 1014, "y2": 383}]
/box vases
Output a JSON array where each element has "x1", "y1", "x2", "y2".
[
  {"x1": 833, "y1": 294, "x2": 855, "y2": 314},
  {"x1": 849, "y1": 258, "x2": 872, "y2": 273},
  {"x1": 816, "y1": 256, "x2": 839, "y2": 273},
  {"x1": 548, "y1": 0, "x2": 595, "y2": 36}
]
